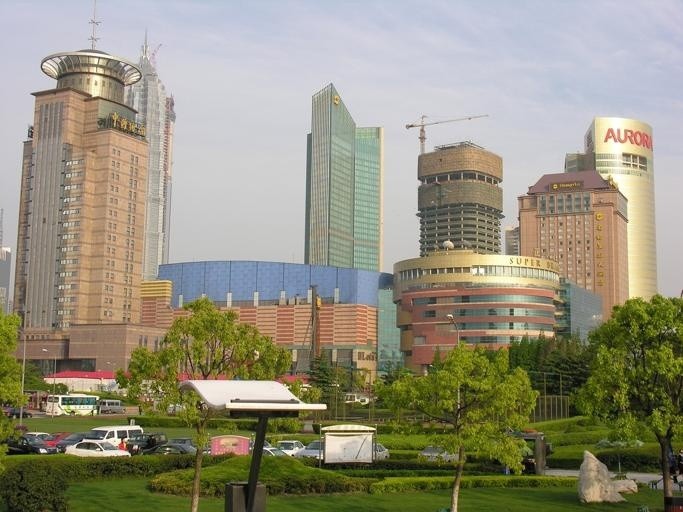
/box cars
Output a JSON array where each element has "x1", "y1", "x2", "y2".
[
  {"x1": 165, "y1": 405, "x2": 175, "y2": 415},
  {"x1": 144, "y1": 391, "x2": 155, "y2": 402},
  {"x1": 3, "y1": 408, "x2": 32, "y2": 420},
  {"x1": 415, "y1": 442, "x2": 467, "y2": 465},
  {"x1": 284, "y1": 382, "x2": 370, "y2": 405}
]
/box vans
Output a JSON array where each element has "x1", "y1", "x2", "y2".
[{"x1": 98, "y1": 398, "x2": 126, "y2": 414}]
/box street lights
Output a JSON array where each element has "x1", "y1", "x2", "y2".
[
  {"x1": 104, "y1": 360, "x2": 114, "y2": 382},
  {"x1": 444, "y1": 312, "x2": 462, "y2": 434},
  {"x1": 40, "y1": 348, "x2": 57, "y2": 419},
  {"x1": 427, "y1": 363, "x2": 438, "y2": 407}
]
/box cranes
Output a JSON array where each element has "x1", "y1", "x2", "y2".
[{"x1": 403, "y1": 110, "x2": 497, "y2": 158}]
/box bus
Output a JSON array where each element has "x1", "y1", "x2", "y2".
[{"x1": 45, "y1": 392, "x2": 100, "y2": 416}]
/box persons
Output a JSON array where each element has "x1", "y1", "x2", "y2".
[
  {"x1": 119, "y1": 437, "x2": 128, "y2": 451},
  {"x1": 147, "y1": 436, "x2": 156, "y2": 449}
]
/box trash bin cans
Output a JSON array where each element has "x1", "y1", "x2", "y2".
[{"x1": 225, "y1": 482, "x2": 266, "y2": 512}]
